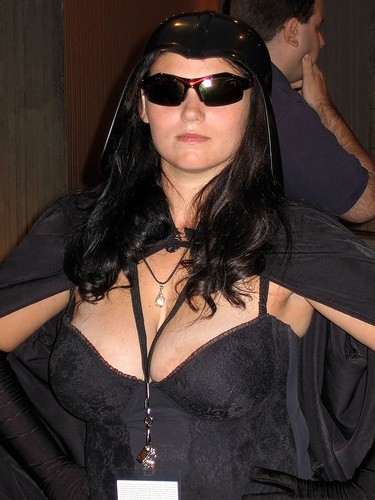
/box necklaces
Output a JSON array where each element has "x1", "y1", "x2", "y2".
[{"x1": 142, "y1": 234, "x2": 190, "y2": 307}]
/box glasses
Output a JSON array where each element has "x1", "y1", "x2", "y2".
[{"x1": 138, "y1": 72, "x2": 253, "y2": 108}]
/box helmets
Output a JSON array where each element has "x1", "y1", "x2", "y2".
[{"x1": 98, "y1": 11, "x2": 285, "y2": 192}]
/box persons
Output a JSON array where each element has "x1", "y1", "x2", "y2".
[
  {"x1": 224, "y1": 1, "x2": 375, "y2": 227},
  {"x1": 0, "y1": 10, "x2": 375, "y2": 500}
]
